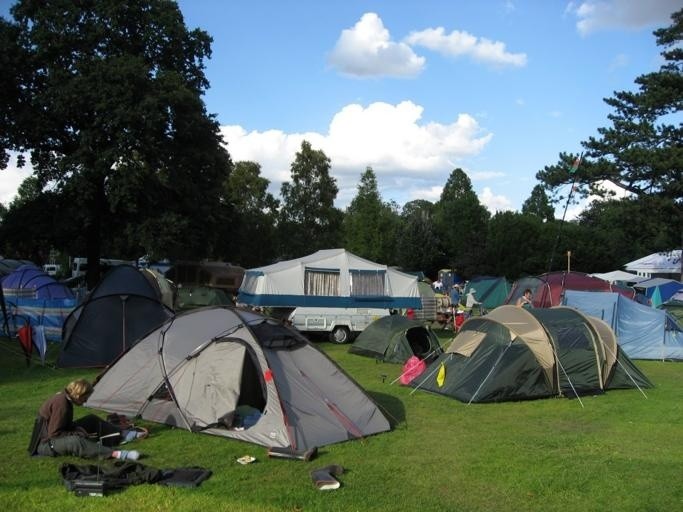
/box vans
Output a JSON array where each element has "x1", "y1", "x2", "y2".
[
  {"x1": 72, "y1": 258, "x2": 120, "y2": 277},
  {"x1": 237, "y1": 249, "x2": 423, "y2": 344}
]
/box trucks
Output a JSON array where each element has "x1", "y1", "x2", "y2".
[{"x1": 41, "y1": 264, "x2": 61, "y2": 275}]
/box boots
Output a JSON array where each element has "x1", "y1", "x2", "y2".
[
  {"x1": 310, "y1": 465, "x2": 345, "y2": 491},
  {"x1": 268, "y1": 446, "x2": 317, "y2": 462}
]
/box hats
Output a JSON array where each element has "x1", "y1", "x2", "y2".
[
  {"x1": 452, "y1": 284, "x2": 460, "y2": 287},
  {"x1": 470, "y1": 288, "x2": 476, "y2": 293}
]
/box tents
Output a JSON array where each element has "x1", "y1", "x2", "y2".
[
  {"x1": 84, "y1": 306, "x2": 399, "y2": 460},
  {"x1": 348, "y1": 248, "x2": 682, "y2": 409},
  {"x1": 1, "y1": 247, "x2": 422, "y2": 369}
]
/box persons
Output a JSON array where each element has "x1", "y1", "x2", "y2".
[{"x1": 26, "y1": 378, "x2": 147, "y2": 462}]
